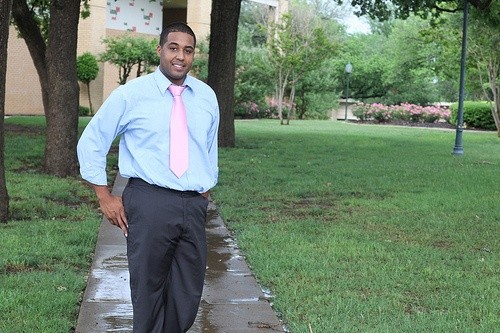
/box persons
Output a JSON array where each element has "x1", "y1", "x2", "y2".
[{"x1": 76, "y1": 23, "x2": 221, "y2": 333}]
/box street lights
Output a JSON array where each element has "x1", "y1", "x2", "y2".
[{"x1": 345, "y1": 63, "x2": 352, "y2": 120}]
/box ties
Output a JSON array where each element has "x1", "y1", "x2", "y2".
[{"x1": 168, "y1": 84, "x2": 190, "y2": 179}]
